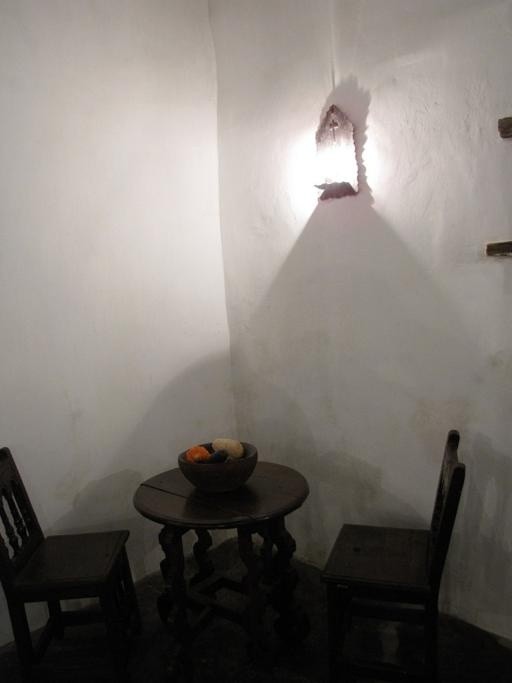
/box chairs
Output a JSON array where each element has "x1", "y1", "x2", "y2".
[
  {"x1": 320, "y1": 429, "x2": 467, "y2": 683},
  {"x1": 0, "y1": 447, "x2": 142, "y2": 683}
]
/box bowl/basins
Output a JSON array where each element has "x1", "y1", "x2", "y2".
[{"x1": 178, "y1": 441, "x2": 257, "y2": 493}]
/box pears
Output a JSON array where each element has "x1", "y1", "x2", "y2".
[{"x1": 212, "y1": 439, "x2": 243, "y2": 457}]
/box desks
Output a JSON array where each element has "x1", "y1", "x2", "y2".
[{"x1": 135, "y1": 461, "x2": 310, "y2": 683}]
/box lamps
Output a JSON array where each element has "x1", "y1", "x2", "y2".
[{"x1": 314, "y1": 105, "x2": 359, "y2": 201}]
